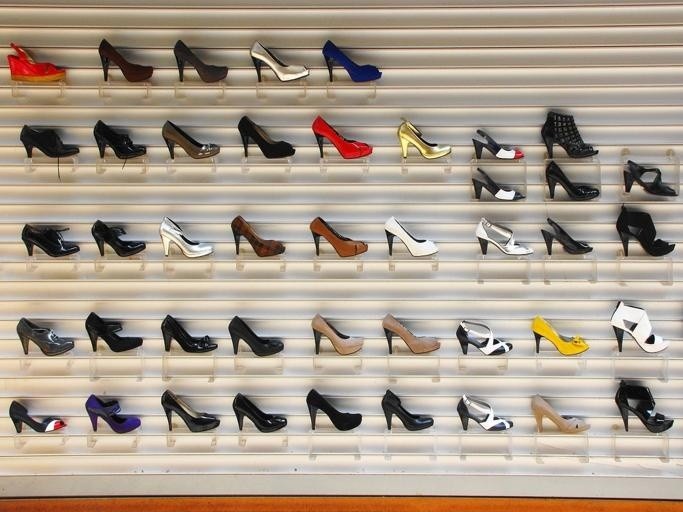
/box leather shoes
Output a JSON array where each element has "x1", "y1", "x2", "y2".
[{"x1": 9, "y1": 43, "x2": 67, "y2": 82}]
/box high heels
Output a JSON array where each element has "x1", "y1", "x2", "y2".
[
  {"x1": 610, "y1": 301, "x2": 669, "y2": 353},
  {"x1": 382, "y1": 313, "x2": 440, "y2": 355},
  {"x1": 251, "y1": 40, "x2": 309, "y2": 82},
  {"x1": 623, "y1": 160, "x2": 678, "y2": 196},
  {"x1": 541, "y1": 112, "x2": 598, "y2": 157},
  {"x1": 310, "y1": 216, "x2": 368, "y2": 257},
  {"x1": 239, "y1": 116, "x2": 296, "y2": 158},
  {"x1": 540, "y1": 218, "x2": 593, "y2": 255},
  {"x1": 312, "y1": 313, "x2": 364, "y2": 355},
  {"x1": 313, "y1": 116, "x2": 372, "y2": 159},
  {"x1": 472, "y1": 130, "x2": 524, "y2": 159},
  {"x1": 162, "y1": 120, "x2": 220, "y2": 159},
  {"x1": 456, "y1": 321, "x2": 513, "y2": 356},
  {"x1": 20, "y1": 124, "x2": 79, "y2": 158},
  {"x1": 381, "y1": 389, "x2": 434, "y2": 431},
  {"x1": 532, "y1": 316, "x2": 589, "y2": 355},
  {"x1": 99, "y1": 38, "x2": 153, "y2": 82},
  {"x1": 616, "y1": 203, "x2": 675, "y2": 257},
  {"x1": 384, "y1": 216, "x2": 440, "y2": 257},
  {"x1": 546, "y1": 160, "x2": 600, "y2": 201},
  {"x1": 531, "y1": 394, "x2": 591, "y2": 434},
  {"x1": 14, "y1": 316, "x2": 286, "y2": 358},
  {"x1": 472, "y1": 168, "x2": 526, "y2": 201},
  {"x1": 174, "y1": 39, "x2": 229, "y2": 82},
  {"x1": 457, "y1": 394, "x2": 513, "y2": 431},
  {"x1": 475, "y1": 217, "x2": 534, "y2": 255},
  {"x1": 398, "y1": 121, "x2": 451, "y2": 159},
  {"x1": 615, "y1": 381, "x2": 674, "y2": 433},
  {"x1": 94, "y1": 119, "x2": 146, "y2": 158},
  {"x1": 307, "y1": 389, "x2": 362, "y2": 431},
  {"x1": 323, "y1": 40, "x2": 381, "y2": 82},
  {"x1": 19, "y1": 219, "x2": 291, "y2": 258},
  {"x1": 8, "y1": 398, "x2": 286, "y2": 433}
]
